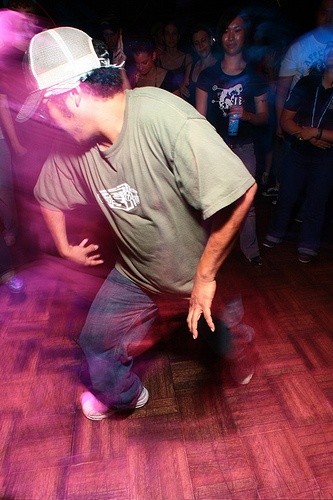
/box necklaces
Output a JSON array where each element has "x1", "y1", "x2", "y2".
[{"x1": 311, "y1": 86, "x2": 333, "y2": 128}]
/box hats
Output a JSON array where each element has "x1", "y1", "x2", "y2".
[{"x1": 14, "y1": 25, "x2": 101, "y2": 122}]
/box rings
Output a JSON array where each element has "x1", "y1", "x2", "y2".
[
  {"x1": 299, "y1": 137, "x2": 301, "y2": 140},
  {"x1": 302, "y1": 138, "x2": 304, "y2": 141}
]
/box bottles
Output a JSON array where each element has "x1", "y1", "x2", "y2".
[{"x1": 228, "y1": 95, "x2": 239, "y2": 137}]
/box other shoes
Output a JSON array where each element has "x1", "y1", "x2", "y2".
[
  {"x1": 248, "y1": 256, "x2": 263, "y2": 269},
  {"x1": 298, "y1": 253, "x2": 312, "y2": 264},
  {"x1": 262, "y1": 240, "x2": 276, "y2": 248},
  {"x1": 82, "y1": 386, "x2": 150, "y2": 420},
  {"x1": 231, "y1": 360, "x2": 255, "y2": 384},
  {"x1": 7, "y1": 275, "x2": 25, "y2": 293}
]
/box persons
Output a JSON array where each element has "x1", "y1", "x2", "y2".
[
  {"x1": 0, "y1": 62, "x2": 29, "y2": 292},
  {"x1": 102, "y1": 8, "x2": 333, "y2": 264},
  {"x1": 15, "y1": 27, "x2": 257, "y2": 420}
]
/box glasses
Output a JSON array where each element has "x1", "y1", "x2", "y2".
[{"x1": 39, "y1": 97, "x2": 51, "y2": 120}]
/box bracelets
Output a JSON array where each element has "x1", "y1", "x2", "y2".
[{"x1": 316, "y1": 128, "x2": 323, "y2": 140}]
